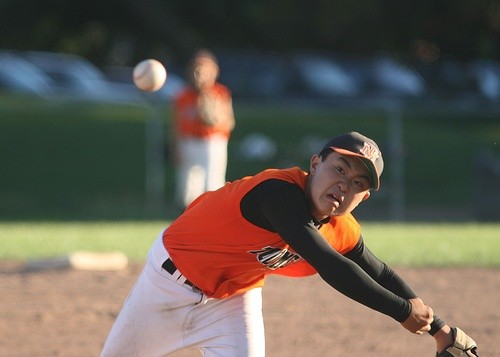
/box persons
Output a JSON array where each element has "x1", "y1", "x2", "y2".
[
  {"x1": 169, "y1": 48, "x2": 236, "y2": 209},
  {"x1": 98, "y1": 130, "x2": 478, "y2": 357}
]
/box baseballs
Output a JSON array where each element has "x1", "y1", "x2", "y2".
[{"x1": 132, "y1": 59, "x2": 167, "y2": 93}]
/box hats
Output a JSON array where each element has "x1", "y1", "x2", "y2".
[{"x1": 322, "y1": 131, "x2": 384, "y2": 192}]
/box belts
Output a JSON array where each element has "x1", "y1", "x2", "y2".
[{"x1": 162, "y1": 258, "x2": 201, "y2": 290}]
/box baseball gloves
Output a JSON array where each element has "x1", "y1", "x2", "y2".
[{"x1": 436, "y1": 327, "x2": 481, "y2": 357}]
